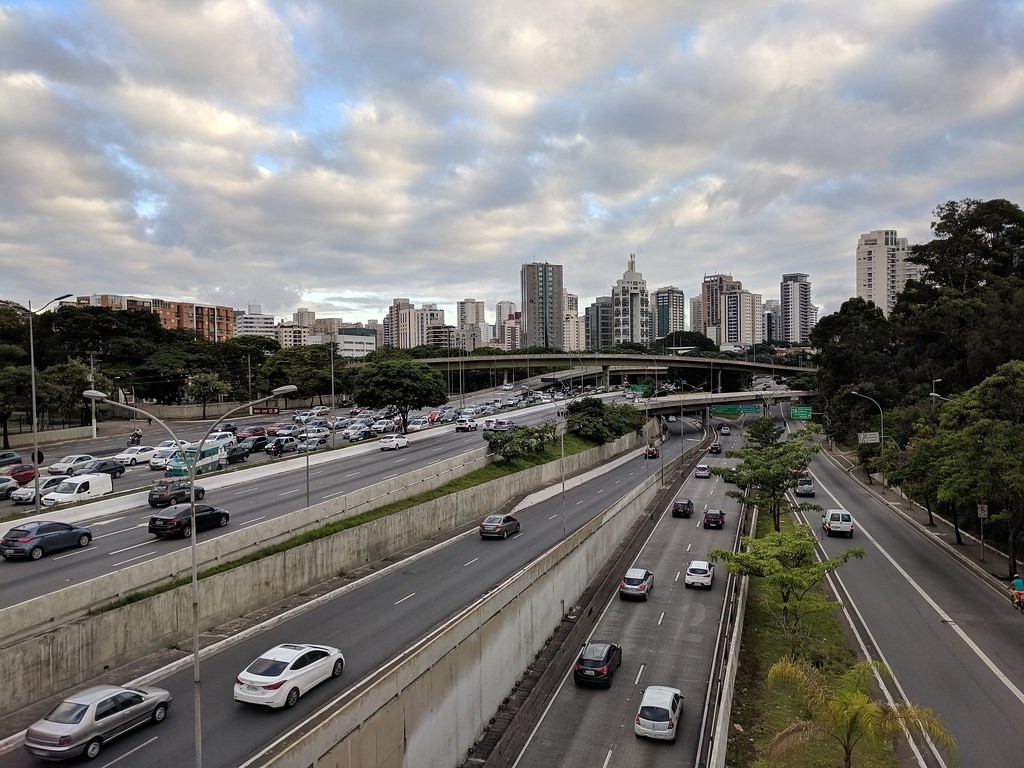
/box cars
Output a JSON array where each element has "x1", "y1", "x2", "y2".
[
  {"x1": 482, "y1": 419, "x2": 498, "y2": 431},
  {"x1": 0, "y1": 464, "x2": 40, "y2": 485},
  {"x1": 0, "y1": 451, "x2": 22, "y2": 468},
  {"x1": 703, "y1": 509, "x2": 727, "y2": 529},
  {"x1": 378, "y1": 433, "x2": 411, "y2": 452},
  {"x1": 0, "y1": 520, "x2": 93, "y2": 560},
  {"x1": 634, "y1": 685, "x2": 685, "y2": 744},
  {"x1": 762, "y1": 374, "x2": 787, "y2": 391},
  {"x1": 0, "y1": 475, "x2": 20, "y2": 500},
  {"x1": 196, "y1": 379, "x2": 636, "y2": 464},
  {"x1": 148, "y1": 449, "x2": 181, "y2": 471},
  {"x1": 147, "y1": 502, "x2": 230, "y2": 539},
  {"x1": 156, "y1": 440, "x2": 191, "y2": 452},
  {"x1": 720, "y1": 426, "x2": 731, "y2": 436},
  {"x1": 644, "y1": 447, "x2": 660, "y2": 459},
  {"x1": 148, "y1": 480, "x2": 206, "y2": 508},
  {"x1": 111, "y1": 445, "x2": 163, "y2": 466},
  {"x1": 233, "y1": 642, "x2": 346, "y2": 712},
  {"x1": 24, "y1": 684, "x2": 174, "y2": 762},
  {"x1": 48, "y1": 454, "x2": 99, "y2": 475},
  {"x1": 668, "y1": 415, "x2": 676, "y2": 422},
  {"x1": 716, "y1": 422, "x2": 726, "y2": 430},
  {"x1": 694, "y1": 464, "x2": 712, "y2": 479},
  {"x1": 10, "y1": 474, "x2": 72, "y2": 505},
  {"x1": 70, "y1": 459, "x2": 126, "y2": 478},
  {"x1": 479, "y1": 514, "x2": 521, "y2": 540},
  {"x1": 708, "y1": 442, "x2": 723, "y2": 454}
]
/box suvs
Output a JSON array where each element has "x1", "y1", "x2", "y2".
[
  {"x1": 684, "y1": 560, "x2": 717, "y2": 591},
  {"x1": 671, "y1": 498, "x2": 695, "y2": 519},
  {"x1": 573, "y1": 640, "x2": 622, "y2": 689},
  {"x1": 620, "y1": 567, "x2": 654, "y2": 602},
  {"x1": 493, "y1": 420, "x2": 515, "y2": 432},
  {"x1": 455, "y1": 417, "x2": 478, "y2": 432},
  {"x1": 793, "y1": 477, "x2": 816, "y2": 497}
]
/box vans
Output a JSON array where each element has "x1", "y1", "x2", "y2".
[
  {"x1": 164, "y1": 443, "x2": 228, "y2": 478},
  {"x1": 821, "y1": 509, "x2": 854, "y2": 539},
  {"x1": 40, "y1": 472, "x2": 114, "y2": 507}
]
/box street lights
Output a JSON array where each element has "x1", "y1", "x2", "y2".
[
  {"x1": 82, "y1": 384, "x2": 298, "y2": 768},
  {"x1": 0, "y1": 292, "x2": 74, "y2": 516},
  {"x1": 851, "y1": 391, "x2": 885, "y2": 494},
  {"x1": 933, "y1": 379, "x2": 942, "y2": 406},
  {"x1": 701, "y1": 353, "x2": 724, "y2": 394},
  {"x1": 582, "y1": 348, "x2": 608, "y2": 393},
  {"x1": 666, "y1": 387, "x2": 703, "y2": 464},
  {"x1": 632, "y1": 390, "x2": 662, "y2": 479}
]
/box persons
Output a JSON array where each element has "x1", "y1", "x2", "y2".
[
  {"x1": 131, "y1": 429, "x2": 138, "y2": 443},
  {"x1": 762, "y1": 384, "x2": 767, "y2": 391},
  {"x1": 148, "y1": 417, "x2": 153, "y2": 426},
  {"x1": 1007, "y1": 574, "x2": 1024, "y2": 603},
  {"x1": 274, "y1": 439, "x2": 281, "y2": 457},
  {"x1": 430, "y1": 412, "x2": 435, "y2": 425}
]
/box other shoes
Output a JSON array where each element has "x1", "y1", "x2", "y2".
[{"x1": 1014, "y1": 600, "x2": 1018, "y2": 603}]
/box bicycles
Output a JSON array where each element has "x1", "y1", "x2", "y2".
[{"x1": 1006, "y1": 588, "x2": 1024, "y2": 615}]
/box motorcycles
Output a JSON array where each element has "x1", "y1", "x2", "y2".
[{"x1": 127, "y1": 433, "x2": 142, "y2": 447}]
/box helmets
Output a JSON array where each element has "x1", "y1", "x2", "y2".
[{"x1": 1014, "y1": 574, "x2": 1019, "y2": 578}]
